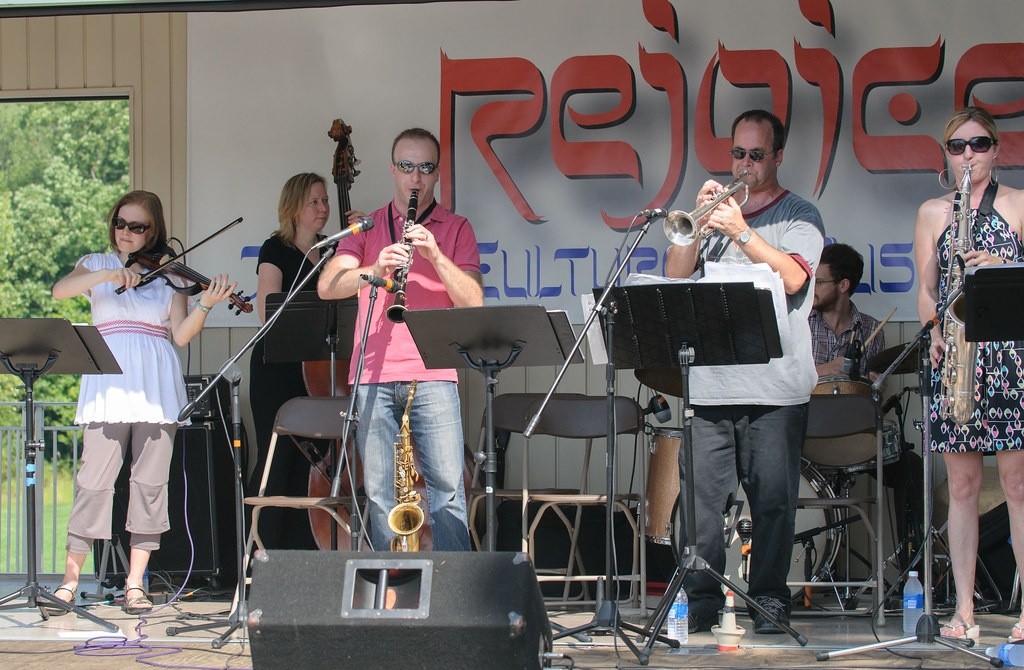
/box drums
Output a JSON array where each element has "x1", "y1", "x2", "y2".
[
  {"x1": 667, "y1": 454, "x2": 843, "y2": 613},
  {"x1": 641, "y1": 426, "x2": 685, "y2": 547},
  {"x1": 800, "y1": 373, "x2": 885, "y2": 468}
]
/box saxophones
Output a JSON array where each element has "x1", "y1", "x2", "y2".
[
  {"x1": 937, "y1": 162, "x2": 980, "y2": 426},
  {"x1": 387, "y1": 378, "x2": 426, "y2": 553}
]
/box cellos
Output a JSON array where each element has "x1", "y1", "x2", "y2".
[{"x1": 300, "y1": 117, "x2": 486, "y2": 559}]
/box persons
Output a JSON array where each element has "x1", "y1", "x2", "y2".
[
  {"x1": 809, "y1": 243, "x2": 941, "y2": 602},
  {"x1": 916, "y1": 107, "x2": 1024, "y2": 642},
  {"x1": 665, "y1": 108, "x2": 825, "y2": 634},
  {"x1": 46, "y1": 191, "x2": 238, "y2": 616},
  {"x1": 251, "y1": 172, "x2": 365, "y2": 548},
  {"x1": 317, "y1": 128, "x2": 484, "y2": 550}
]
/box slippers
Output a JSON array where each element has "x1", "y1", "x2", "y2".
[
  {"x1": 940, "y1": 613, "x2": 981, "y2": 638},
  {"x1": 1007, "y1": 623, "x2": 1024, "y2": 643}
]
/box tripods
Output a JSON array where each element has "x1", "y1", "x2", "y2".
[
  {"x1": 0, "y1": 316, "x2": 120, "y2": 632},
  {"x1": 524, "y1": 218, "x2": 680, "y2": 666},
  {"x1": 812, "y1": 281, "x2": 1004, "y2": 669},
  {"x1": 166, "y1": 243, "x2": 334, "y2": 650},
  {"x1": 589, "y1": 281, "x2": 807, "y2": 656}
]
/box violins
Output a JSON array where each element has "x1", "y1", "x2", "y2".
[{"x1": 131, "y1": 243, "x2": 253, "y2": 316}]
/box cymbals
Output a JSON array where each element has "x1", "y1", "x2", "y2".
[{"x1": 866, "y1": 341, "x2": 928, "y2": 375}]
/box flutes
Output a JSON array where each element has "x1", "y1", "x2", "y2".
[{"x1": 385, "y1": 188, "x2": 420, "y2": 323}]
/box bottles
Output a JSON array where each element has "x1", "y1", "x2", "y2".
[
  {"x1": 985, "y1": 644, "x2": 1024, "y2": 668},
  {"x1": 903, "y1": 571, "x2": 923, "y2": 633},
  {"x1": 667, "y1": 587, "x2": 688, "y2": 646}
]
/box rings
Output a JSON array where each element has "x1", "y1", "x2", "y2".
[{"x1": 424, "y1": 235, "x2": 428, "y2": 241}]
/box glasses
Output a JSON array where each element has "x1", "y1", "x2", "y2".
[
  {"x1": 112, "y1": 216, "x2": 151, "y2": 234},
  {"x1": 393, "y1": 160, "x2": 439, "y2": 174},
  {"x1": 729, "y1": 148, "x2": 774, "y2": 162},
  {"x1": 815, "y1": 278, "x2": 837, "y2": 287},
  {"x1": 947, "y1": 136, "x2": 997, "y2": 155}
]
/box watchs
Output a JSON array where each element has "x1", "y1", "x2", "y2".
[
  {"x1": 194, "y1": 299, "x2": 213, "y2": 314},
  {"x1": 735, "y1": 226, "x2": 754, "y2": 246},
  {"x1": 999, "y1": 256, "x2": 1006, "y2": 265}
]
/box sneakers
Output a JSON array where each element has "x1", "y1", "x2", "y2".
[
  {"x1": 659, "y1": 609, "x2": 721, "y2": 634},
  {"x1": 751, "y1": 595, "x2": 789, "y2": 633}
]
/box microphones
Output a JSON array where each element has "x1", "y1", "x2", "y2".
[
  {"x1": 841, "y1": 321, "x2": 856, "y2": 373},
  {"x1": 649, "y1": 395, "x2": 671, "y2": 424},
  {"x1": 359, "y1": 274, "x2": 400, "y2": 293},
  {"x1": 637, "y1": 208, "x2": 668, "y2": 218},
  {"x1": 315, "y1": 218, "x2": 374, "y2": 249},
  {"x1": 880, "y1": 387, "x2": 907, "y2": 418}
]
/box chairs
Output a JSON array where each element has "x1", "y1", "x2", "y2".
[
  {"x1": 224, "y1": 395, "x2": 370, "y2": 622},
  {"x1": 461, "y1": 392, "x2": 653, "y2": 621},
  {"x1": 783, "y1": 393, "x2": 888, "y2": 627}
]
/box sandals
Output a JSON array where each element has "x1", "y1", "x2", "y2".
[
  {"x1": 122, "y1": 585, "x2": 154, "y2": 614},
  {"x1": 42, "y1": 584, "x2": 77, "y2": 615}
]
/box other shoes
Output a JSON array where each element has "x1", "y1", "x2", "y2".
[{"x1": 922, "y1": 574, "x2": 946, "y2": 602}]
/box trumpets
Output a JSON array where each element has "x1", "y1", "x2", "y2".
[{"x1": 662, "y1": 169, "x2": 751, "y2": 247}]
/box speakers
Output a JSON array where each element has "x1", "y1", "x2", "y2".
[
  {"x1": 91, "y1": 424, "x2": 240, "y2": 585},
  {"x1": 245, "y1": 550, "x2": 553, "y2": 669}
]
